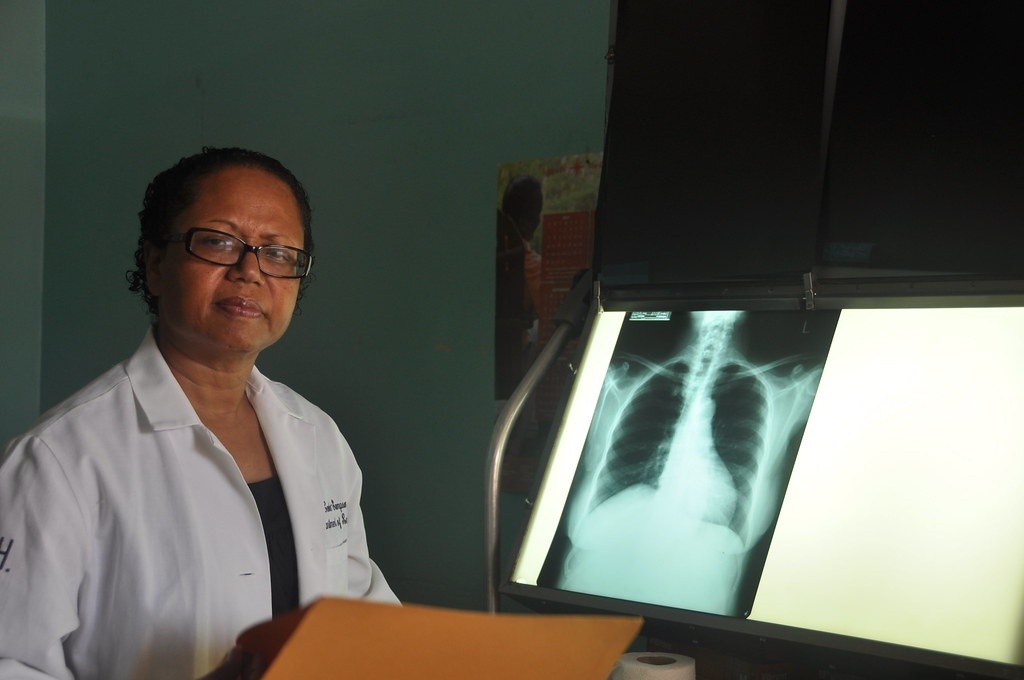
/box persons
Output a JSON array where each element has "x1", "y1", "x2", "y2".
[{"x1": 0, "y1": 145, "x2": 401, "y2": 680}]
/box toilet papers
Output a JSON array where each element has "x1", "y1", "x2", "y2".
[{"x1": 612, "y1": 651, "x2": 695, "y2": 679}]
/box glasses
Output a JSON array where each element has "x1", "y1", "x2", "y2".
[{"x1": 163, "y1": 227, "x2": 312, "y2": 278}]
